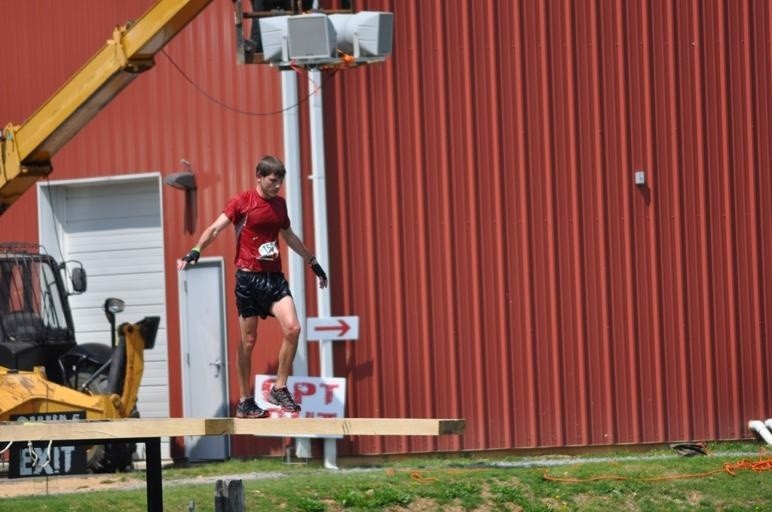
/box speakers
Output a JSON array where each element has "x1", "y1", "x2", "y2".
[{"x1": 258, "y1": 10, "x2": 393, "y2": 62}]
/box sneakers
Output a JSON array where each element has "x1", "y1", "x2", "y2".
[
  {"x1": 268, "y1": 385, "x2": 301, "y2": 412},
  {"x1": 236, "y1": 398, "x2": 269, "y2": 418}
]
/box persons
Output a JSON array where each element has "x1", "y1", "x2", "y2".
[{"x1": 175, "y1": 154, "x2": 329, "y2": 420}]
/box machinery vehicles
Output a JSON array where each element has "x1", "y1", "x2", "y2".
[{"x1": 0, "y1": 0, "x2": 395, "y2": 474}]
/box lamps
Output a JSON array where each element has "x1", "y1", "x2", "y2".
[{"x1": 167, "y1": 172, "x2": 198, "y2": 192}]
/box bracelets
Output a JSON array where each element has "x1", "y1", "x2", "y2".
[{"x1": 308, "y1": 256, "x2": 316, "y2": 265}]
